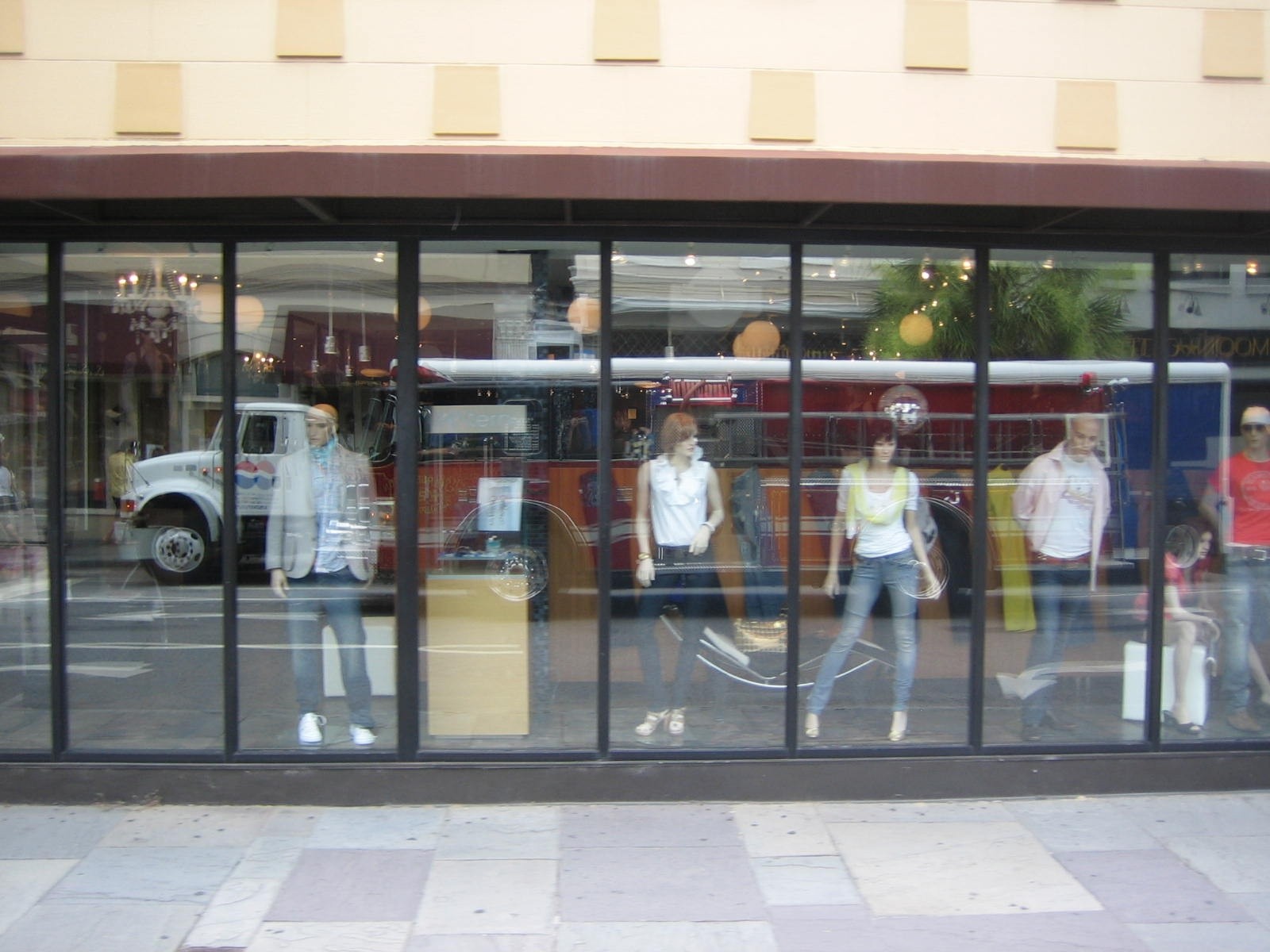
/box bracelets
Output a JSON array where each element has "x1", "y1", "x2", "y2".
[{"x1": 699, "y1": 522, "x2": 715, "y2": 533}]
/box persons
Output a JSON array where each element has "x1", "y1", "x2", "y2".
[
  {"x1": 1129, "y1": 514, "x2": 1220, "y2": 734},
  {"x1": 98, "y1": 440, "x2": 138, "y2": 545},
  {"x1": 635, "y1": 413, "x2": 725, "y2": 737},
  {"x1": 1199, "y1": 406, "x2": 1270, "y2": 733},
  {"x1": 264, "y1": 404, "x2": 382, "y2": 747},
  {"x1": 0, "y1": 459, "x2": 25, "y2": 550},
  {"x1": 1012, "y1": 412, "x2": 1112, "y2": 741},
  {"x1": 804, "y1": 420, "x2": 942, "y2": 743}
]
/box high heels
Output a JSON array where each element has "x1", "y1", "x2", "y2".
[
  {"x1": 889, "y1": 712, "x2": 909, "y2": 740},
  {"x1": 804, "y1": 712, "x2": 820, "y2": 738},
  {"x1": 636, "y1": 708, "x2": 670, "y2": 737},
  {"x1": 1163, "y1": 710, "x2": 1202, "y2": 734},
  {"x1": 1252, "y1": 699, "x2": 1270, "y2": 718},
  {"x1": 669, "y1": 707, "x2": 687, "y2": 734}
]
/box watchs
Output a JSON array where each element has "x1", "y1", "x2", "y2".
[{"x1": 637, "y1": 552, "x2": 652, "y2": 561}]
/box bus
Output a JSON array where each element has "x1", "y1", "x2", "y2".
[{"x1": 348, "y1": 353, "x2": 1235, "y2": 626}]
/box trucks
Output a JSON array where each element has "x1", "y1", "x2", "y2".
[{"x1": 107, "y1": 400, "x2": 315, "y2": 588}]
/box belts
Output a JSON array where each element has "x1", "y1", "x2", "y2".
[
  {"x1": 1032, "y1": 553, "x2": 1091, "y2": 565},
  {"x1": 1219, "y1": 545, "x2": 1270, "y2": 561}
]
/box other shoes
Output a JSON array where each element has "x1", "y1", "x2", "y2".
[
  {"x1": 1225, "y1": 706, "x2": 1262, "y2": 733},
  {"x1": 1021, "y1": 722, "x2": 1043, "y2": 740},
  {"x1": 1039, "y1": 710, "x2": 1080, "y2": 730}
]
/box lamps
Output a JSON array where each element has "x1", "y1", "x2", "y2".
[
  {"x1": 108, "y1": 253, "x2": 263, "y2": 344},
  {"x1": 1186, "y1": 293, "x2": 1195, "y2": 313},
  {"x1": 393, "y1": 294, "x2": 432, "y2": 332},
  {"x1": 898, "y1": 286, "x2": 934, "y2": 347},
  {"x1": 731, "y1": 310, "x2": 782, "y2": 360},
  {"x1": 567, "y1": 258, "x2": 602, "y2": 334}
]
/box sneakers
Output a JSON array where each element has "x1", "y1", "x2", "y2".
[
  {"x1": 297, "y1": 711, "x2": 327, "y2": 743},
  {"x1": 349, "y1": 722, "x2": 376, "y2": 744}
]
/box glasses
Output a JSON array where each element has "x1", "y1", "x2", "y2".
[{"x1": 1242, "y1": 424, "x2": 1270, "y2": 434}]
